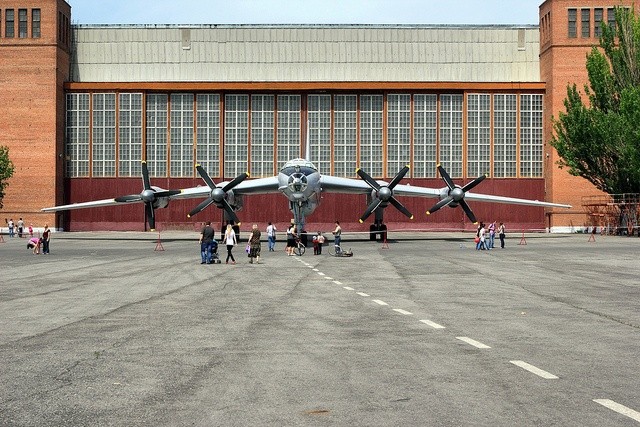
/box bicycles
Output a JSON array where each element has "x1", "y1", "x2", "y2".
[
  {"x1": 328, "y1": 245, "x2": 354, "y2": 257},
  {"x1": 293, "y1": 237, "x2": 305, "y2": 256}
]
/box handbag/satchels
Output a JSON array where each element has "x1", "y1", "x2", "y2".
[
  {"x1": 272, "y1": 236, "x2": 276, "y2": 242},
  {"x1": 245, "y1": 244, "x2": 250, "y2": 255},
  {"x1": 503, "y1": 234, "x2": 505, "y2": 238}
]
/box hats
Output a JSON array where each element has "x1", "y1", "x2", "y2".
[{"x1": 312, "y1": 236, "x2": 316, "y2": 240}]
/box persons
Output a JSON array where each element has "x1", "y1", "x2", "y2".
[
  {"x1": 28, "y1": 224, "x2": 34, "y2": 237},
  {"x1": 313, "y1": 236, "x2": 319, "y2": 256},
  {"x1": 331, "y1": 220, "x2": 342, "y2": 253},
  {"x1": 497, "y1": 222, "x2": 505, "y2": 248},
  {"x1": 7, "y1": 218, "x2": 14, "y2": 237},
  {"x1": 14, "y1": 224, "x2": 18, "y2": 234},
  {"x1": 248, "y1": 224, "x2": 261, "y2": 264},
  {"x1": 488, "y1": 219, "x2": 497, "y2": 249},
  {"x1": 475, "y1": 221, "x2": 489, "y2": 250},
  {"x1": 286, "y1": 223, "x2": 297, "y2": 256},
  {"x1": 27, "y1": 237, "x2": 42, "y2": 255},
  {"x1": 317, "y1": 230, "x2": 325, "y2": 255},
  {"x1": 265, "y1": 221, "x2": 276, "y2": 252},
  {"x1": 200, "y1": 220, "x2": 214, "y2": 264},
  {"x1": 42, "y1": 224, "x2": 51, "y2": 254},
  {"x1": 223, "y1": 224, "x2": 238, "y2": 265},
  {"x1": 18, "y1": 217, "x2": 25, "y2": 237}
]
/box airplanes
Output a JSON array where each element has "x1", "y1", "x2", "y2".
[{"x1": 41, "y1": 157, "x2": 572, "y2": 245}]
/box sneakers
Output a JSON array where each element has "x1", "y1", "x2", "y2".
[
  {"x1": 36, "y1": 251, "x2": 39, "y2": 254},
  {"x1": 200, "y1": 261, "x2": 206, "y2": 264},
  {"x1": 290, "y1": 253, "x2": 296, "y2": 256},
  {"x1": 499, "y1": 246, "x2": 504, "y2": 249},
  {"x1": 47, "y1": 252, "x2": 49, "y2": 255},
  {"x1": 232, "y1": 261, "x2": 235, "y2": 264},
  {"x1": 226, "y1": 261, "x2": 228, "y2": 263},
  {"x1": 32, "y1": 252, "x2": 35, "y2": 254},
  {"x1": 256, "y1": 261, "x2": 258, "y2": 264},
  {"x1": 42, "y1": 252, "x2": 45, "y2": 254},
  {"x1": 207, "y1": 262, "x2": 210, "y2": 264},
  {"x1": 288, "y1": 254, "x2": 291, "y2": 256},
  {"x1": 271, "y1": 247, "x2": 274, "y2": 251},
  {"x1": 489, "y1": 248, "x2": 492, "y2": 249},
  {"x1": 248, "y1": 261, "x2": 253, "y2": 264},
  {"x1": 492, "y1": 246, "x2": 495, "y2": 248}
]
